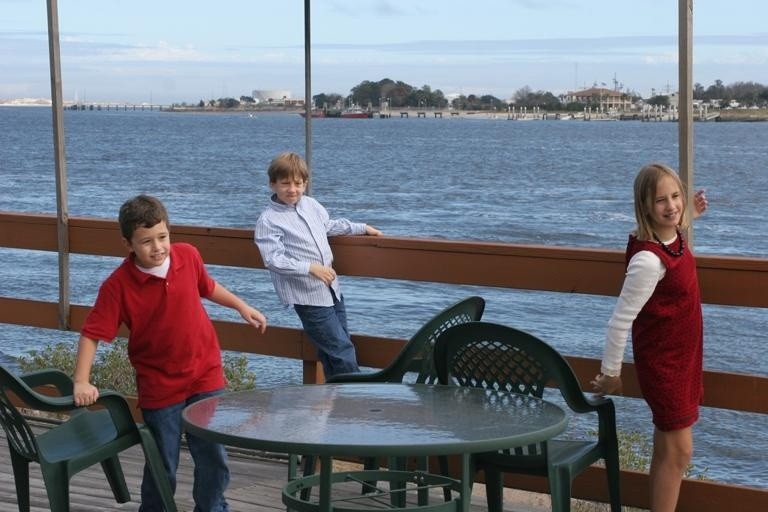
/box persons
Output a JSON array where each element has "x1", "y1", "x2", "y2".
[
  {"x1": 73, "y1": 194, "x2": 268, "y2": 512},
  {"x1": 588, "y1": 161, "x2": 708, "y2": 511},
  {"x1": 253, "y1": 151, "x2": 382, "y2": 381}
]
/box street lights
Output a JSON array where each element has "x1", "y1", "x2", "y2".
[{"x1": 387, "y1": 95, "x2": 391, "y2": 112}]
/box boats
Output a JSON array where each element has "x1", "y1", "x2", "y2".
[{"x1": 297, "y1": 99, "x2": 374, "y2": 119}]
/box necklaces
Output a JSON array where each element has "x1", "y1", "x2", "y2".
[{"x1": 651, "y1": 227, "x2": 685, "y2": 256}]
